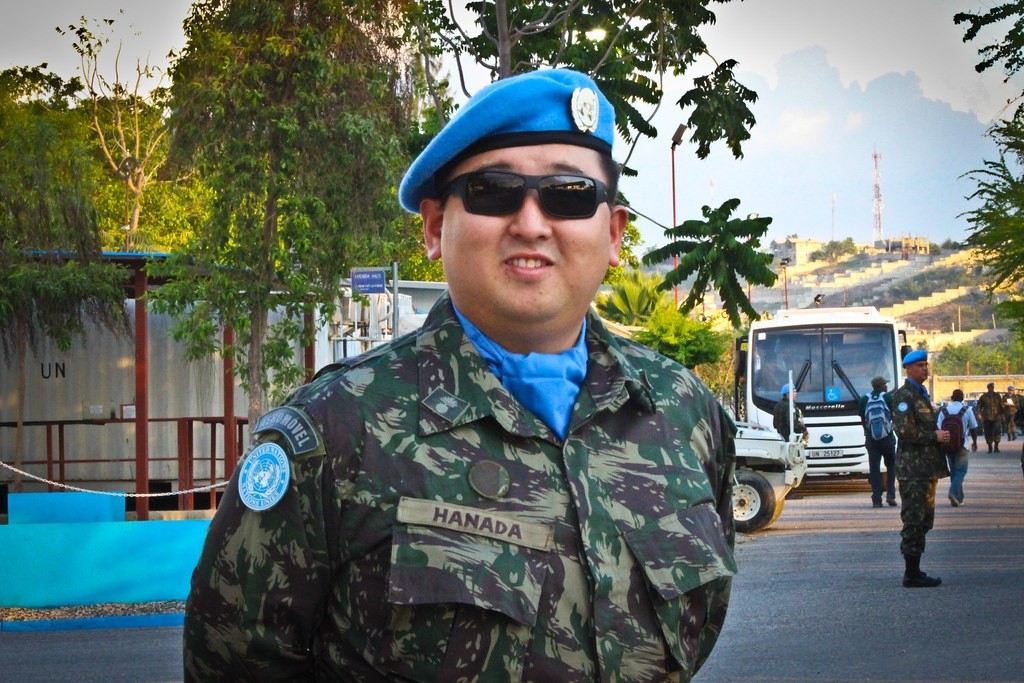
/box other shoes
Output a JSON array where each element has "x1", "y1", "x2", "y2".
[
  {"x1": 873, "y1": 499, "x2": 897, "y2": 508},
  {"x1": 785, "y1": 493, "x2": 803, "y2": 499}
]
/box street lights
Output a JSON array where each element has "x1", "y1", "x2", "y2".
[{"x1": 671, "y1": 121, "x2": 689, "y2": 319}]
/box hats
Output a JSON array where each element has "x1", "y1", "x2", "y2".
[
  {"x1": 871, "y1": 377, "x2": 890, "y2": 387},
  {"x1": 781, "y1": 384, "x2": 795, "y2": 394},
  {"x1": 903, "y1": 350, "x2": 927, "y2": 367},
  {"x1": 987, "y1": 383, "x2": 993, "y2": 386},
  {"x1": 1008, "y1": 386, "x2": 1014, "y2": 389},
  {"x1": 399, "y1": 69, "x2": 614, "y2": 213}
]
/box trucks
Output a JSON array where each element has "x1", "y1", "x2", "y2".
[{"x1": 734, "y1": 305, "x2": 913, "y2": 495}]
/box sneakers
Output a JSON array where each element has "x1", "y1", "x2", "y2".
[{"x1": 949, "y1": 494, "x2": 966, "y2": 507}]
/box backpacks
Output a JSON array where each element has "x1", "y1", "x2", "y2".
[{"x1": 941, "y1": 406, "x2": 968, "y2": 455}]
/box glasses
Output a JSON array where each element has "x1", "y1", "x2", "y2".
[{"x1": 441, "y1": 170, "x2": 614, "y2": 219}]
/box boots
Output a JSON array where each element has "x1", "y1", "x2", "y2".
[
  {"x1": 988, "y1": 442, "x2": 1000, "y2": 453},
  {"x1": 1008, "y1": 432, "x2": 1016, "y2": 441},
  {"x1": 904, "y1": 554, "x2": 942, "y2": 587}
]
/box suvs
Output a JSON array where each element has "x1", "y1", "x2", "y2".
[{"x1": 727, "y1": 421, "x2": 808, "y2": 534}]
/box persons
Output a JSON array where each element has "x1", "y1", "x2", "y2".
[
  {"x1": 937, "y1": 389, "x2": 978, "y2": 507},
  {"x1": 926, "y1": 383, "x2": 1024, "y2": 453},
  {"x1": 894, "y1": 350, "x2": 950, "y2": 587},
  {"x1": 773, "y1": 384, "x2": 807, "y2": 500},
  {"x1": 182, "y1": 69, "x2": 738, "y2": 683},
  {"x1": 858, "y1": 377, "x2": 899, "y2": 508}
]
